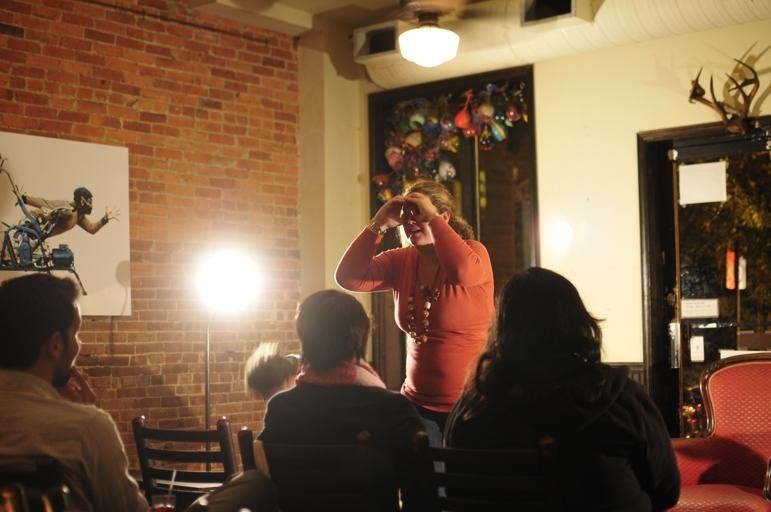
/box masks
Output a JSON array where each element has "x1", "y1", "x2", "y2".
[{"x1": 80, "y1": 198, "x2": 92, "y2": 215}]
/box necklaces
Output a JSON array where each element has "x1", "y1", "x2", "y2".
[{"x1": 406, "y1": 254, "x2": 443, "y2": 344}]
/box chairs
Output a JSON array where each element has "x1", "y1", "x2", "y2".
[
  {"x1": 0, "y1": 451, "x2": 100, "y2": 512},
  {"x1": 237, "y1": 430, "x2": 400, "y2": 511},
  {"x1": 417, "y1": 433, "x2": 565, "y2": 511},
  {"x1": 664, "y1": 353, "x2": 771, "y2": 512},
  {"x1": 131, "y1": 414, "x2": 237, "y2": 507}
]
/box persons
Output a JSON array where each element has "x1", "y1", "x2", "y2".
[
  {"x1": 441, "y1": 267, "x2": 682, "y2": 512},
  {"x1": 245, "y1": 341, "x2": 387, "y2": 403},
  {"x1": 259, "y1": 288, "x2": 444, "y2": 509},
  {"x1": 11, "y1": 183, "x2": 122, "y2": 261},
  {"x1": 333, "y1": 182, "x2": 495, "y2": 449},
  {"x1": 0, "y1": 273, "x2": 279, "y2": 512}
]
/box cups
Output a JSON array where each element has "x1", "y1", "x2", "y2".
[{"x1": 152, "y1": 494, "x2": 177, "y2": 512}]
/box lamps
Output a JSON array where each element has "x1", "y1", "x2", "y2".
[{"x1": 398, "y1": 10, "x2": 460, "y2": 68}]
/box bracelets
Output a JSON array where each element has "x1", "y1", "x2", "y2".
[{"x1": 369, "y1": 220, "x2": 388, "y2": 235}]
